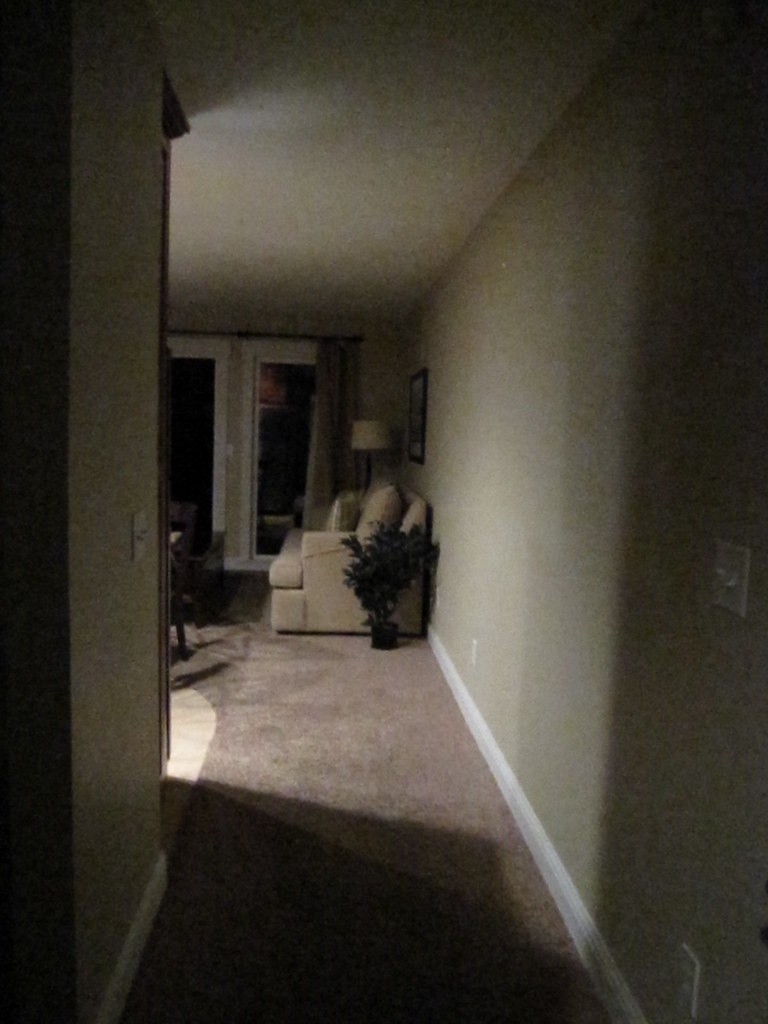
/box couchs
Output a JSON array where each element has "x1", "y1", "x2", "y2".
[{"x1": 268, "y1": 480, "x2": 426, "y2": 635}]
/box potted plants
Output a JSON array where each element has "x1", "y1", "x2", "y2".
[{"x1": 335, "y1": 520, "x2": 441, "y2": 648}]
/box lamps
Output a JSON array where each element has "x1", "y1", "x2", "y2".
[{"x1": 350, "y1": 420, "x2": 395, "y2": 490}]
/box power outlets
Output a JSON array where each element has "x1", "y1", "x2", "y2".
[{"x1": 678, "y1": 942, "x2": 700, "y2": 1020}]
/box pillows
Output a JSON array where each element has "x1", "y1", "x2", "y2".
[
  {"x1": 356, "y1": 486, "x2": 402, "y2": 551},
  {"x1": 360, "y1": 465, "x2": 399, "y2": 512},
  {"x1": 325, "y1": 490, "x2": 358, "y2": 534}
]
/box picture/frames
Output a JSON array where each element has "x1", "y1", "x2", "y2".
[{"x1": 408, "y1": 368, "x2": 429, "y2": 465}]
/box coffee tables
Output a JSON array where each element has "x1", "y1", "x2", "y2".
[{"x1": 172, "y1": 530, "x2": 227, "y2": 625}]
